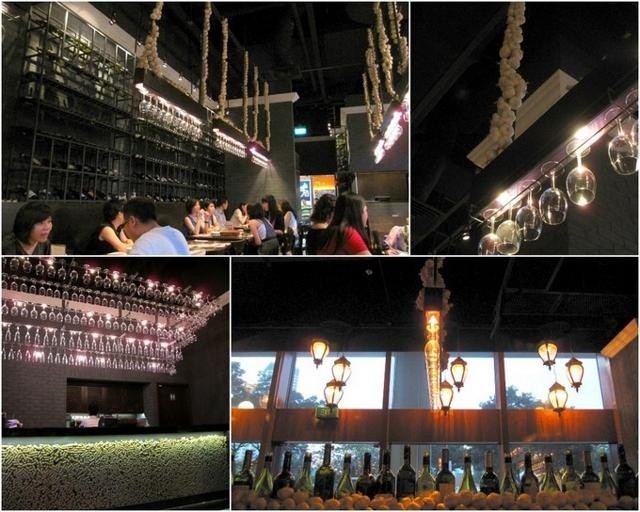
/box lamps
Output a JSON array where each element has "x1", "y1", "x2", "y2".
[
  {"x1": 535, "y1": 295, "x2": 584, "y2": 417},
  {"x1": 307, "y1": 334, "x2": 352, "y2": 408},
  {"x1": 423, "y1": 257, "x2": 467, "y2": 417}
]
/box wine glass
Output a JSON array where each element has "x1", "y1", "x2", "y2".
[
  {"x1": 2, "y1": 257, "x2": 224, "y2": 376},
  {"x1": 137, "y1": 90, "x2": 270, "y2": 169},
  {"x1": 475, "y1": 87, "x2": 638, "y2": 255}
]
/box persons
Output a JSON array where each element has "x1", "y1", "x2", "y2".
[
  {"x1": 91, "y1": 195, "x2": 409, "y2": 254},
  {"x1": 1, "y1": 200, "x2": 54, "y2": 257},
  {"x1": 78, "y1": 405, "x2": 100, "y2": 428}
]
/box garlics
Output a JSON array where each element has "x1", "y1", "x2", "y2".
[
  {"x1": 361, "y1": 2, "x2": 408, "y2": 139},
  {"x1": 490, "y1": 2, "x2": 527, "y2": 147},
  {"x1": 145, "y1": 2, "x2": 271, "y2": 153}
]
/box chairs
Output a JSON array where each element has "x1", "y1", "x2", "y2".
[{"x1": 256, "y1": 223, "x2": 307, "y2": 257}]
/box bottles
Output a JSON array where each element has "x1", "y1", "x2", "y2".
[
  {"x1": 232, "y1": 442, "x2": 638, "y2": 502},
  {"x1": 25, "y1": 154, "x2": 215, "y2": 203}
]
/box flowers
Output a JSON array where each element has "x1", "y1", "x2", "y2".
[{"x1": 187, "y1": 227, "x2": 255, "y2": 255}]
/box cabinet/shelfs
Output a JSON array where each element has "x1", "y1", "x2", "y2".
[{"x1": 66, "y1": 384, "x2": 143, "y2": 413}]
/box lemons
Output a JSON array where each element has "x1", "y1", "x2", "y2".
[{"x1": 232, "y1": 485, "x2": 638, "y2": 510}]
